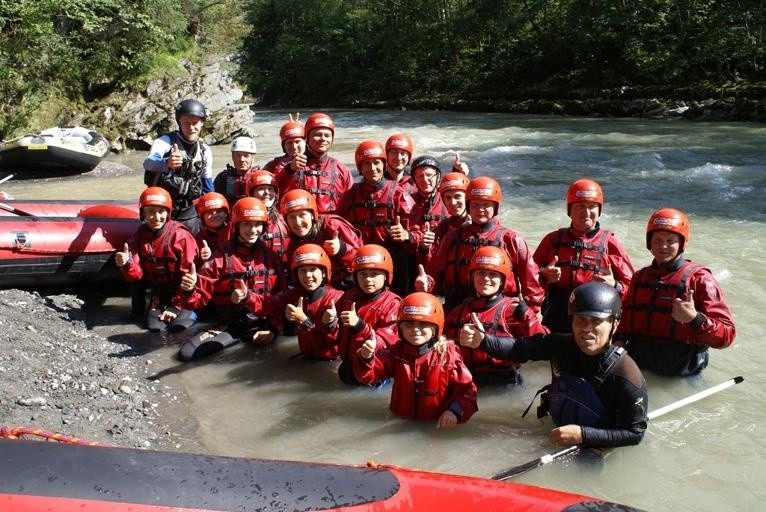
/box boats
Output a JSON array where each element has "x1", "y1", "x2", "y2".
[
  {"x1": 0, "y1": 424, "x2": 639, "y2": 512},
  {"x1": 0, "y1": 124, "x2": 111, "y2": 172},
  {"x1": 0, "y1": 198, "x2": 147, "y2": 295}
]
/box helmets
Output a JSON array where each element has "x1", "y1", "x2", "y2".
[
  {"x1": 565, "y1": 179, "x2": 603, "y2": 216},
  {"x1": 140, "y1": 171, "x2": 317, "y2": 232},
  {"x1": 568, "y1": 281, "x2": 622, "y2": 320},
  {"x1": 355, "y1": 133, "x2": 502, "y2": 214},
  {"x1": 291, "y1": 244, "x2": 444, "y2": 340},
  {"x1": 231, "y1": 137, "x2": 256, "y2": 154},
  {"x1": 647, "y1": 208, "x2": 689, "y2": 252},
  {"x1": 176, "y1": 100, "x2": 205, "y2": 121},
  {"x1": 280, "y1": 113, "x2": 334, "y2": 141},
  {"x1": 468, "y1": 245, "x2": 509, "y2": 290}
]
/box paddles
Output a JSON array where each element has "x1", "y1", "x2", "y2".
[{"x1": 489, "y1": 376, "x2": 746, "y2": 483}]
